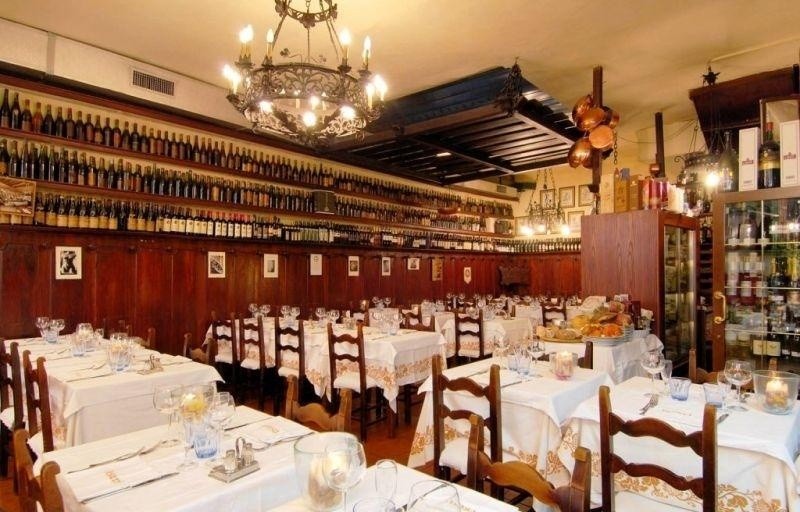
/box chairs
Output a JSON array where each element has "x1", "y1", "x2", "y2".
[{"x1": 0, "y1": 294, "x2": 800, "y2": 512}]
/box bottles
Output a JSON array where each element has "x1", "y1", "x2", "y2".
[
  {"x1": 0, "y1": 87, "x2": 517, "y2": 255},
  {"x1": 726, "y1": 204, "x2": 800, "y2": 374},
  {"x1": 755, "y1": 121, "x2": 781, "y2": 190},
  {"x1": 716, "y1": 129, "x2": 739, "y2": 193}
]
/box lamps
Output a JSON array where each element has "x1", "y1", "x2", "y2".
[
  {"x1": 516, "y1": 165, "x2": 573, "y2": 237},
  {"x1": 221, "y1": 0, "x2": 393, "y2": 151},
  {"x1": 668, "y1": 69, "x2": 736, "y2": 209}
]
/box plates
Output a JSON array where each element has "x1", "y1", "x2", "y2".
[{"x1": 540, "y1": 324, "x2": 637, "y2": 346}]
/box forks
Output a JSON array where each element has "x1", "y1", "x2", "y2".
[{"x1": 59, "y1": 438, "x2": 180, "y2": 505}]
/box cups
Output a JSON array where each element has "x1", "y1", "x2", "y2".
[{"x1": 752, "y1": 370, "x2": 800, "y2": 416}]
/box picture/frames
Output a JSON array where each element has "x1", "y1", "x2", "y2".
[
  {"x1": 514, "y1": 183, "x2": 598, "y2": 237},
  {"x1": 309, "y1": 253, "x2": 324, "y2": 277},
  {"x1": 208, "y1": 251, "x2": 225, "y2": 278},
  {"x1": 53, "y1": 243, "x2": 85, "y2": 284},
  {"x1": 264, "y1": 253, "x2": 279, "y2": 278}
]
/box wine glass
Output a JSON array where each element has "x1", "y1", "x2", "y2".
[
  {"x1": 485, "y1": 326, "x2": 580, "y2": 381},
  {"x1": 638, "y1": 347, "x2": 753, "y2": 418},
  {"x1": 372, "y1": 292, "x2": 578, "y2": 327},
  {"x1": 151, "y1": 377, "x2": 464, "y2": 511},
  {"x1": 246, "y1": 302, "x2": 405, "y2": 342},
  {"x1": 34, "y1": 316, "x2": 141, "y2": 375}
]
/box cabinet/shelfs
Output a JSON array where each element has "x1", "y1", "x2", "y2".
[
  {"x1": 578, "y1": 206, "x2": 700, "y2": 378},
  {"x1": 710, "y1": 185, "x2": 800, "y2": 401},
  {"x1": 1, "y1": 72, "x2": 518, "y2": 254}
]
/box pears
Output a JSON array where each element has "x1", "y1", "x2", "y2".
[{"x1": 572, "y1": 314, "x2": 588, "y2": 328}]
